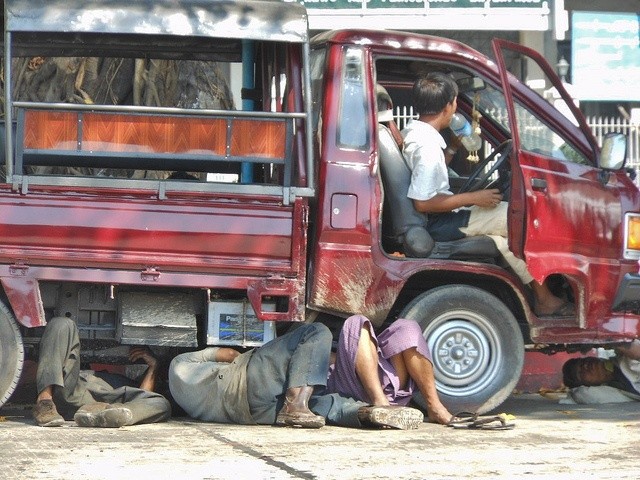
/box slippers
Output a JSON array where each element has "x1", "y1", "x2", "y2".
[
  {"x1": 454, "y1": 415, "x2": 515, "y2": 430},
  {"x1": 448, "y1": 412, "x2": 479, "y2": 427},
  {"x1": 535, "y1": 299, "x2": 576, "y2": 318}
]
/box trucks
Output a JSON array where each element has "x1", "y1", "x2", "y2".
[{"x1": 0, "y1": 0, "x2": 640, "y2": 418}]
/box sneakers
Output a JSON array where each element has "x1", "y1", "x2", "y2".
[
  {"x1": 32, "y1": 399, "x2": 65, "y2": 427},
  {"x1": 74, "y1": 403, "x2": 132, "y2": 428}
]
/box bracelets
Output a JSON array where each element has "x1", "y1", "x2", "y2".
[{"x1": 446, "y1": 147, "x2": 457, "y2": 156}]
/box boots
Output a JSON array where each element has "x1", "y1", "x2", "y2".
[
  {"x1": 359, "y1": 404, "x2": 425, "y2": 430},
  {"x1": 276, "y1": 386, "x2": 326, "y2": 428}
]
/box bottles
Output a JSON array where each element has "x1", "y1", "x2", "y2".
[{"x1": 448, "y1": 112, "x2": 483, "y2": 152}]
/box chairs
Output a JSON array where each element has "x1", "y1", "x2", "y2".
[{"x1": 377, "y1": 83, "x2": 499, "y2": 261}]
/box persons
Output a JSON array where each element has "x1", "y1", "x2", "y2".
[
  {"x1": 168, "y1": 322, "x2": 424, "y2": 430},
  {"x1": 31, "y1": 315, "x2": 173, "y2": 428},
  {"x1": 321, "y1": 314, "x2": 462, "y2": 429},
  {"x1": 562, "y1": 341, "x2": 640, "y2": 405},
  {"x1": 398, "y1": 72, "x2": 580, "y2": 317}
]
